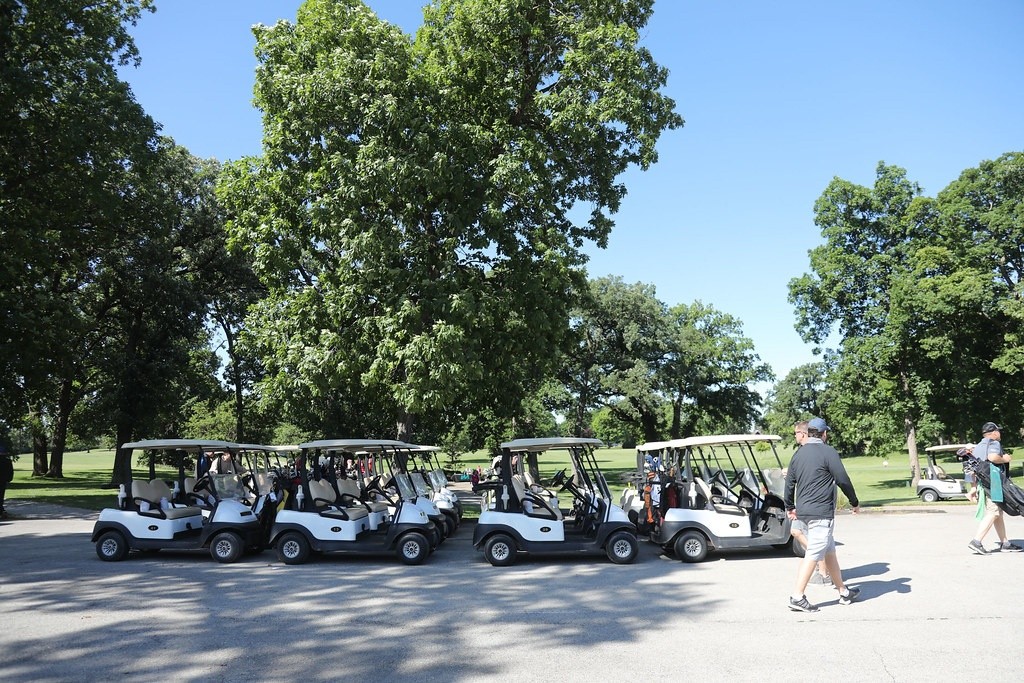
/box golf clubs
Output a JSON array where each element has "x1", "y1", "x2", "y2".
[{"x1": 286, "y1": 458, "x2": 293, "y2": 478}]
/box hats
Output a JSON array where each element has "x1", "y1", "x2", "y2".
[
  {"x1": 982, "y1": 422, "x2": 1003, "y2": 434},
  {"x1": 808, "y1": 418, "x2": 831, "y2": 433},
  {"x1": 966, "y1": 443, "x2": 977, "y2": 449}
]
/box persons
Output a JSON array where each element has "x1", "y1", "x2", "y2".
[
  {"x1": 0, "y1": 448, "x2": 13, "y2": 520},
  {"x1": 789, "y1": 421, "x2": 833, "y2": 586},
  {"x1": 968, "y1": 421, "x2": 1024, "y2": 556},
  {"x1": 201, "y1": 450, "x2": 670, "y2": 526},
  {"x1": 962, "y1": 443, "x2": 978, "y2": 505},
  {"x1": 784, "y1": 420, "x2": 861, "y2": 613}
]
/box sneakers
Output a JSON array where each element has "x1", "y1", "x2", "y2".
[
  {"x1": 808, "y1": 573, "x2": 833, "y2": 585},
  {"x1": 787, "y1": 594, "x2": 820, "y2": 612},
  {"x1": 968, "y1": 540, "x2": 987, "y2": 555},
  {"x1": 971, "y1": 498, "x2": 978, "y2": 502},
  {"x1": 1000, "y1": 542, "x2": 1023, "y2": 552},
  {"x1": 839, "y1": 585, "x2": 861, "y2": 605},
  {"x1": 965, "y1": 493, "x2": 972, "y2": 501}
]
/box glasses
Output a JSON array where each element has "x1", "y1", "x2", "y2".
[{"x1": 794, "y1": 431, "x2": 807, "y2": 435}]
